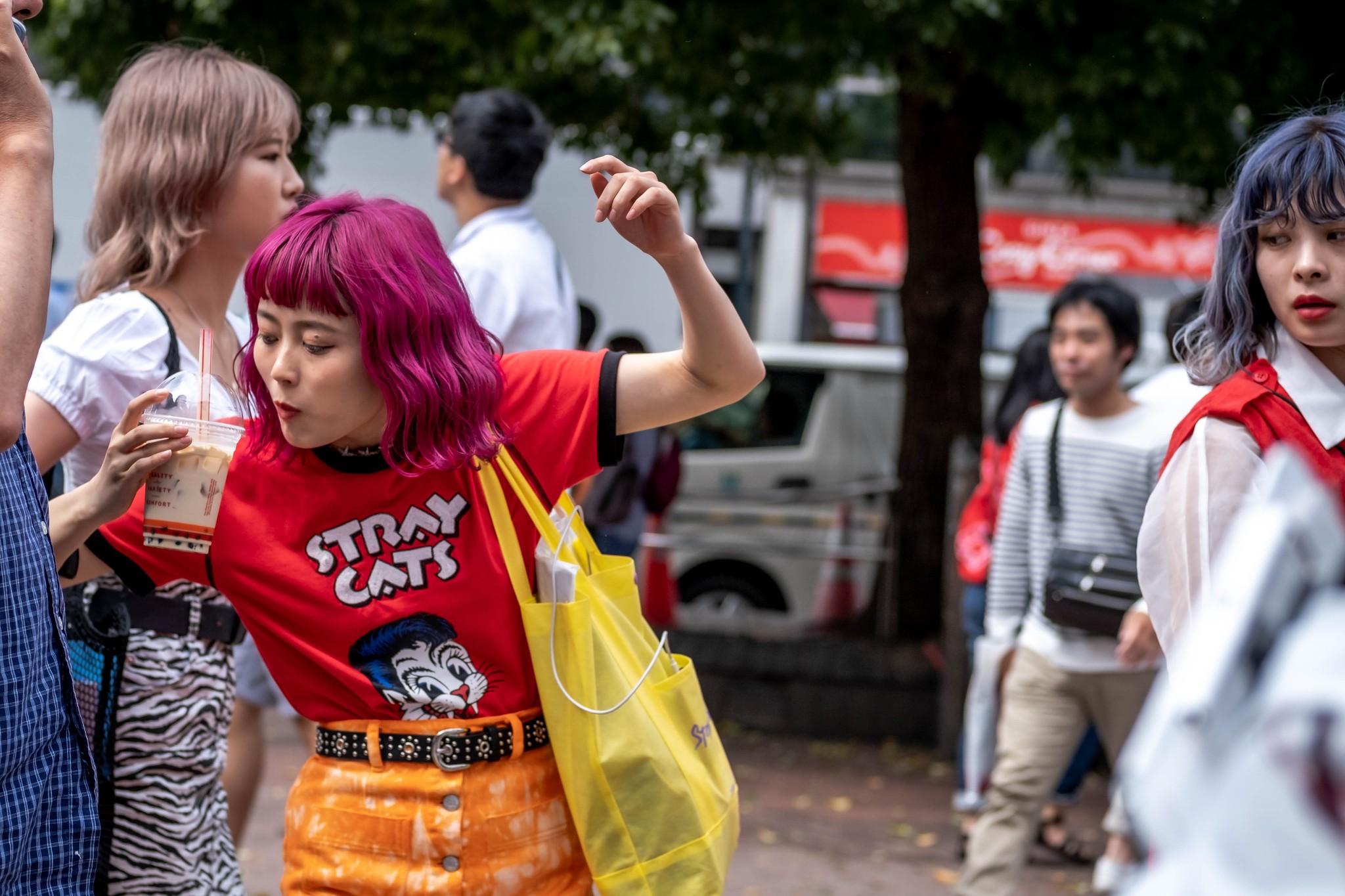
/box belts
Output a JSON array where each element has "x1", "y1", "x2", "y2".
[
  {"x1": 100, "y1": 587, "x2": 248, "y2": 646},
  {"x1": 314, "y1": 714, "x2": 550, "y2": 774}
]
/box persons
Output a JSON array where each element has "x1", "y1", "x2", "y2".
[
  {"x1": 960, "y1": 281, "x2": 1243, "y2": 890},
  {"x1": 570, "y1": 301, "x2": 678, "y2": 560},
  {"x1": 0, "y1": 1, "x2": 101, "y2": 896},
  {"x1": 1134, "y1": 107, "x2": 1344, "y2": 830},
  {"x1": 213, "y1": 92, "x2": 605, "y2": 852},
  {"x1": 19, "y1": 41, "x2": 323, "y2": 896},
  {"x1": 949, "y1": 276, "x2": 1174, "y2": 896},
  {"x1": 44, "y1": 156, "x2": 766, "y2": 896}
]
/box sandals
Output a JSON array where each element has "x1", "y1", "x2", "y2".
[{"x1": 1037, "y1": 813, "x2": 1097, "y2": 864}]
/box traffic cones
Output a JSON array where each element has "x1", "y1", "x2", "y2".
[
  {"x1": 638, "y1": 514, "x2": 684, "y2": 628},
  {"x1": 811, "y1": 498, "x2": 856, "y2": 637}
]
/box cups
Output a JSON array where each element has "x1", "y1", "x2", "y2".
[{"x1": 134, "y1": 410, "x2": 245, "y2": 555}]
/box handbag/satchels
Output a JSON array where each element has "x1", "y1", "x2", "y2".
[
  {"x1": 474, "y1": 423, "x2": 740, "y2": 896},
  {"x1": 1044, "y1": 548, "x2": 1139, "y2": 635},
  {"x1": 58, "y1": 578, "x2": 130, "y2": 896}
]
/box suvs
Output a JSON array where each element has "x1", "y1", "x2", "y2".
[{"x1": 629, "y1": 344, "x2": 1018, "y2": 628}]
